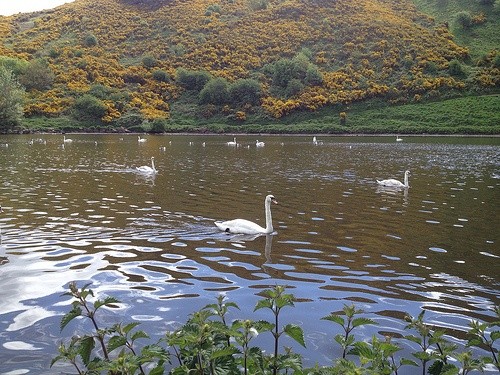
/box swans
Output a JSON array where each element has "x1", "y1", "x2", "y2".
[
  {"x1": 227, "y1": 138, "x2": 237, "y2": 145},
  {"x1": 313, "y1": 137, "x2": 317, "y2": 144},
  {"x1": 63, "y1": 136, "x2": 73, "y2": 143},
  {"x1": 376, "y1": 170, "x2": 411, "y2": 188},
  {"x1": 213, "y1": 194, "x2": 278, "y2": 234},
  {"x1": 255, "y1": 140, "x2": 265, "y2": 146},
  {"x1": 395, "y1": 134, "x2": 403, "y2": 142},
  {"x1": 136, "y1": 156, "x2": 157, "y2": 173},
  {"x1": 137, "y1": 136, "x2": 146, "y2": 142}
]
